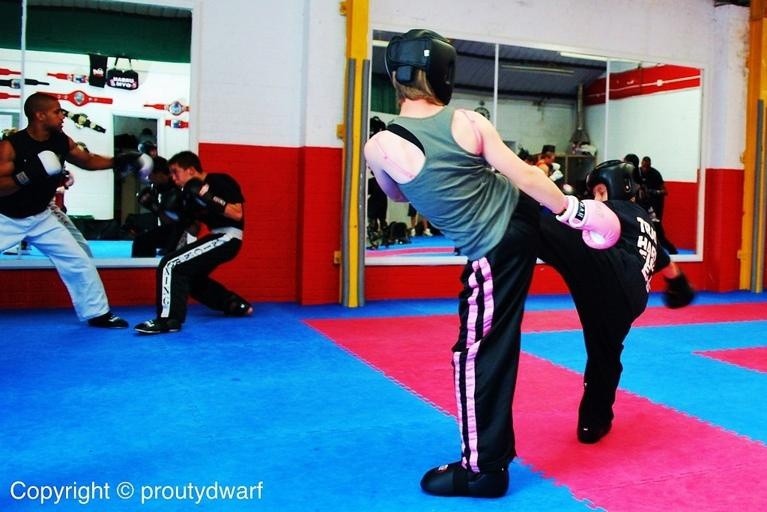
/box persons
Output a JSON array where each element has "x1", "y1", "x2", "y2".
[
  {"x1": 47, "y1": 170, "x2": 94, "y2": 258},
  {"x1": 133, "y1": 152, "x2": 253, "y2": 336},
  {"x1": 0, "y1": 92, "x2": 137, "y2": 331},
  {"x1": 138, "y1": 141, "x2": 157, "y2": 158},
  {"x1": 359, "y1": 26, "x2": 622, "y2": 497},
  {"x1": 532, "y1": 156, "x2": 699, "y2": 444},
  {"x1": 122, "y1": 157, "x2": 184, "y2": 259},
  {"x1": 364, "y1": 117, "x2": 671, "y2": 254}
]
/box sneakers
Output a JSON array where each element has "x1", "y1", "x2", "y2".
[
  {"x1": 576, "y1": 413, "x2": 614, "y2": 443},
  {"x1": 133, "y1": 316, "x2": 182, "y2": 334},
  {"x1": 88, "y1": 310, "x2": 130, "y2": 329},
  {"x1": 224, "y1": 298, "x2": 254, "y2": 317},
  {"x1": 420, "y1": 459, "x2": 508, "y2": 497}
]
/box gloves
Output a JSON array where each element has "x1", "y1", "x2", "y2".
[
  {"x1": 135, "y1": 187, "x2": 162, "y2": 217},
  {"x1": 12, "y1": 150, "x2": 62, "y2": 189},
  {"x1": 112, "y1": 150, "x2": 155, "y2": 181},
  {"x1": 663, "y1": 268, "x2": 694, "y2": 310},
  {"x1": 183, "y1": 177, "x2": 216, "y2": 210},
  {"x1": 163, "y1": 193, "x2": 190, "y2": 229},
  {"x1": 555, "y1": 195, "x2": 622, "y2": 252}
]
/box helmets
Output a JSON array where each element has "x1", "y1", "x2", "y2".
[
  {"x1": 384, "y1": 27, "x2": 458, "y2": 106},
  {"x1": 585, "y1": 159, "x2": 647, "y2": 203}
]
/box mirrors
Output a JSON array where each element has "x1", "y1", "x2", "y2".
[
  {"x1": 365, "y1": 29, "x2": 707, "y2": 263},
  {"x1": 0, "y1": 0, "x2": 199, "y2": 270}
]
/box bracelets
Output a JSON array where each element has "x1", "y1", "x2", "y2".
[{"x1": 205, "y1": 190, "x2": 228, "y2": 214}]
